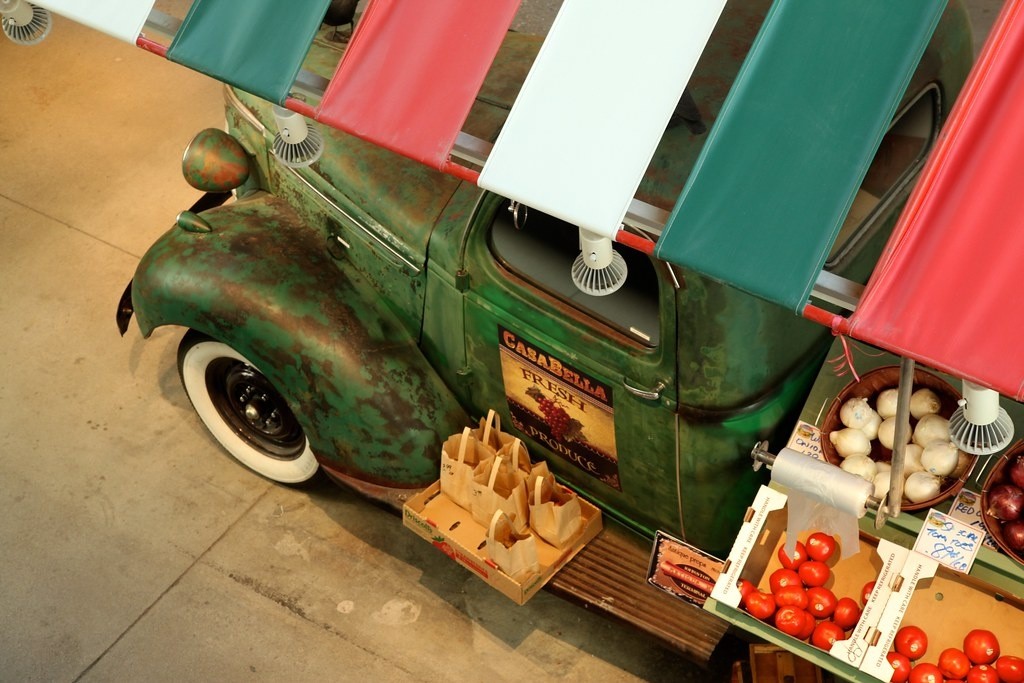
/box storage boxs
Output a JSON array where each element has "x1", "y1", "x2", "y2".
[
  {"x1": 708, "y1": 483, "x2": 1024, "y2": 683},
  {"x1": 403, "y1": 477, "x2": 603, "y2": 606}
]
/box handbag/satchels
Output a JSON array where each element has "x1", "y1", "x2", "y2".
[
  {"x1": 485, "y1": 509, "x2": 537, "y2": 582},
  {"x1": 439, "y1": 409, "x2": 549, "y2": 532},
  {"x1": 528, "y1": 473, "x2": 583, "y2": 549}
]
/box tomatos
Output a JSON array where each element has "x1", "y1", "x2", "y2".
[
  {"x1": 737, "y1": 532, "x2": 878, "y2": 650},
  {"x1": 886, "y1": 625, "x2": 1024, "y2": 683}
]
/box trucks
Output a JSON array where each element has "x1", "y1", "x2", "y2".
[{"x1": 113, "y1": 1, "x2": 1024, "y2": 682}]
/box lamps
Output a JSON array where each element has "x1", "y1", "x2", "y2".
[
  {"x1": 0, "y1": 0, "x2": 52, "y2": 46},
  {"x1": 947, "y1": 378, "x2": 1015, "y2": 456},
  {"x1": 272, "y1": 106, "x2": 324, "y2": 168},
  {"x1": 570, "y1": 227, "x2": 628, "y2": 297}
]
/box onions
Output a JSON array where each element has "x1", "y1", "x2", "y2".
[
  {"x1": 1004, "y1": 520, "x2": 1024, "y2": 550},
  {"x1": 989, "y1": 485, "x2": 1024, "y2": 520},
  {"x1": 1011, "y1": 455, "x2": 1024, "y2": 487}
]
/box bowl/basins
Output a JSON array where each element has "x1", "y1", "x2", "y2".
[{"x1": 821, "y1": 365, "x2": 977, "y2": 517}]
